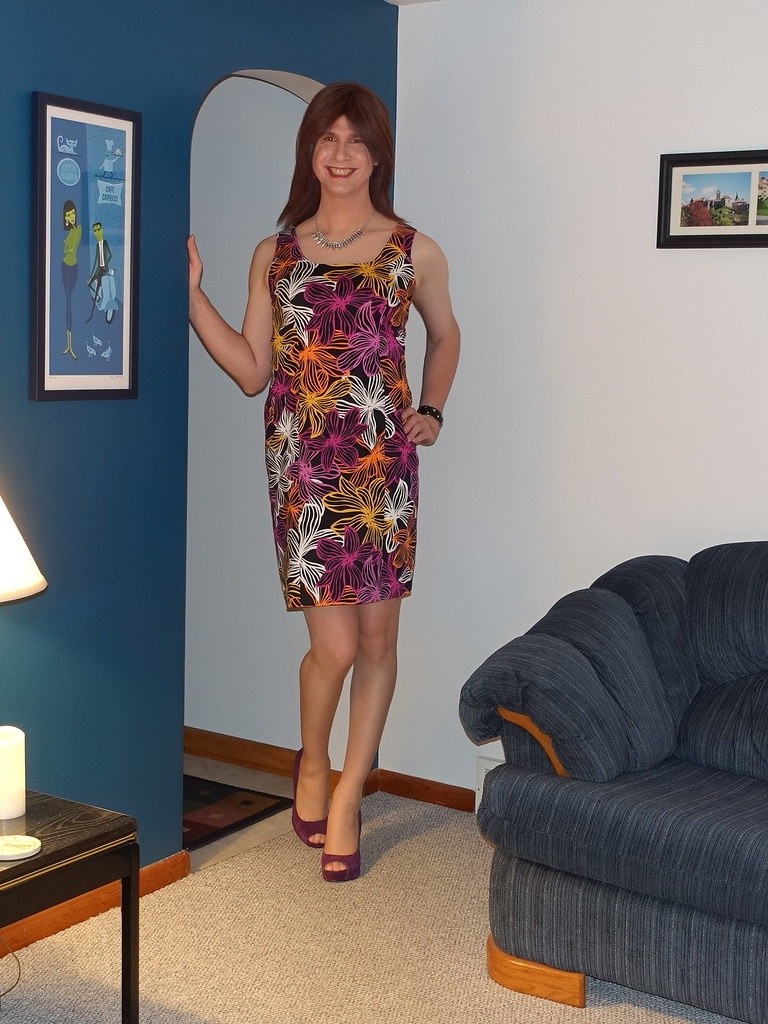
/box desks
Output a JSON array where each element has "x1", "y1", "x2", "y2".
[{"x1": 0, "y1": 789, "x2": 140, "y2": 1024}]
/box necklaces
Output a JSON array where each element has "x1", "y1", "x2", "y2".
[{"x1": 312, "y1": 207, "x2": 375, "y2": 250}]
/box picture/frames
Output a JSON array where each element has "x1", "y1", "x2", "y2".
[
  {"x1": 656, "y1": 149, "x2": 768, "y2": 249},
  {"x1": 29, "y1": 90, "x2": 141, "y2": 402}
]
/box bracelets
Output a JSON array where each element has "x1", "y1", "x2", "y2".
[{"x1": 415, "y1": 405, "x2": 443, "y2": 429}]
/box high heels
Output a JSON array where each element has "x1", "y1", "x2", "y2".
[
  {"x1": 321, "y1": 802, "x2": 363, "y2": 882},
  {"x1": 292, "y1": 742, "x2": 329, "y2": 848}
]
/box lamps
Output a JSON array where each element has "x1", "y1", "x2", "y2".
[{"x1": 1, "y1": 498, "x2": 51, "y2": 862}]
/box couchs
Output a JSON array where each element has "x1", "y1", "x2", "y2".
[{"x1": 459, "y1": 540, "x2": 768, "y2": 1021}]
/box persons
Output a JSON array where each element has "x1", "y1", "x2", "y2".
[{"x1": 186, "y1": 82, "x2": 461, "y2": 884}]
